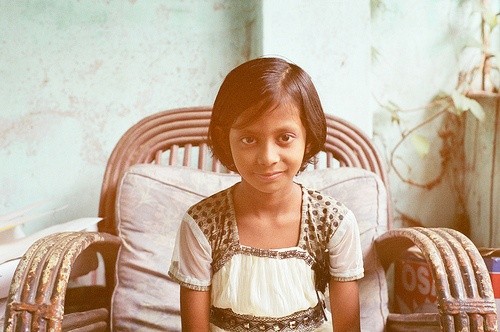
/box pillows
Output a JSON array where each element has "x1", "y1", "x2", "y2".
[{"x1": 110, "y1": 164, "x2": 389, "y2": 332}]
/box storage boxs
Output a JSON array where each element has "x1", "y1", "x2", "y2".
[{"x1": 393, "y1": 247, "x2": 500, "y2": 329}]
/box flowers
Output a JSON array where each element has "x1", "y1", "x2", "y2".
[{"x1": 374, "y1": 0, "x2": 500, "y2": 160}]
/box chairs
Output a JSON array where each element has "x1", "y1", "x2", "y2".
[{"x1": 2, "y1": 105, "x2": 498, "y2": 332}]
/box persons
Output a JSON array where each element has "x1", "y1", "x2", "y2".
[{"x1": 167, "y1": 57, "x2": 365, "y2": 332}]
